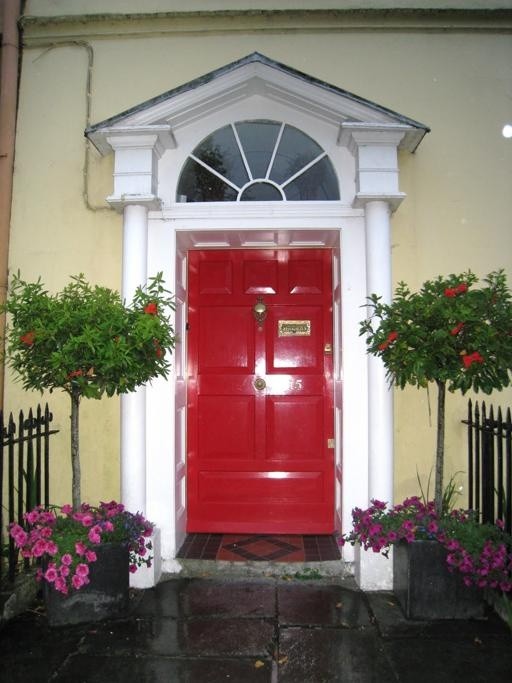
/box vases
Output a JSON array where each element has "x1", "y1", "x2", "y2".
[
  {"x1": 393, "y1": 537, "x2": 484, "y2": 620},
  {"x1": 40, "y1": 543, "x2": 129, "y2": 626}
]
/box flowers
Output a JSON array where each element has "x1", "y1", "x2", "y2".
[
  {"x1": 334, "y1": 269, "x2": 512, "y2": 592},
  {"x1": 0, "y1": 267, "x2": 178, "y2": 594}
]
